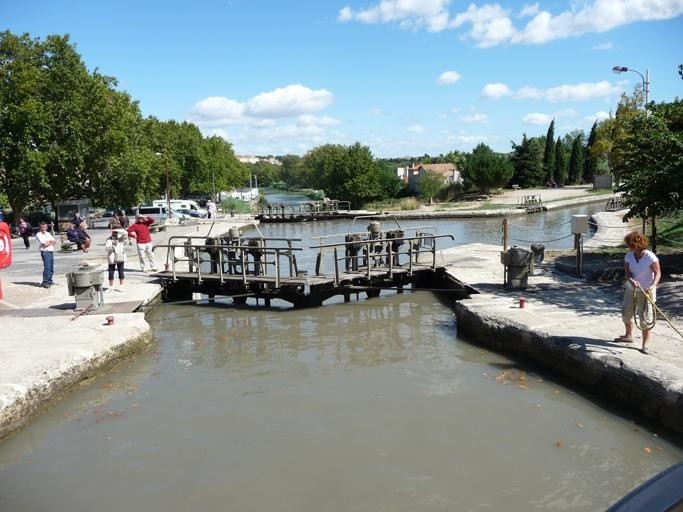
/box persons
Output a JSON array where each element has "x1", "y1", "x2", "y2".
[
  {"x1": 66, "y1": 222, "x2": 91, "y2": 254},
  {"x1": 617, "y1": 231, "x2": 661, "y2": 355},
  {"x1": 72, "y1": 211, "x2": 91, "y2": 242},
  {"x1": 34, "y1": 220, "x2": 59, "y2": 288},
  {"x1": 128, "y1": 214, "x2": 159, "y2": 271},
  {"x1": 119, "y1": 210, "x2": 128, "y2": 233},
  {"x1": 17, "y1": 216, "x2": 31, "y2": 250},
  {"x1": 104, "y1": 226, "x2": 128, "y2": 286}
]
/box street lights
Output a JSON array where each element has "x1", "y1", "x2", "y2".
[
  {"x1": 154, "y1": 151, "x2": 171, "y2": 218},
  {"x1": 613, "y1": 66, "x2": 650, "y2": 234}
]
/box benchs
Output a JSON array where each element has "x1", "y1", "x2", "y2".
[{"x1": 59, "y1": 232, "x2": 78, "y2": 252}]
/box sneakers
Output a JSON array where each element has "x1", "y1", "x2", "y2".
[
  {"x1": 642, "y1": 346, "x2": 649, "y2": 353},
  {"x1": 614, "y1": 336, "x2": 632, "y2": 343}
]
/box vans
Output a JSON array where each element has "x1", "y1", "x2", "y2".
[
  {"x1": 136, "y1": 205, "x2": 189, "y2": 220},
  {"x1": 152, "y1": 199, "x2": 208, "y2": 218}
]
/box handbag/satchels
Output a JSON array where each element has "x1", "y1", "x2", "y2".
[{"x1": 114, "y1": 253, "x2": 124, "y2": 262}]
